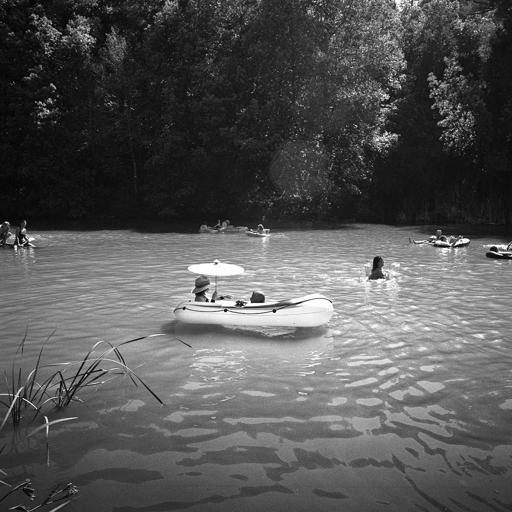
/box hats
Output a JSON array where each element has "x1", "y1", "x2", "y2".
[{"x1": 192, "y1": 275, "x2": 211, "y2": 293}]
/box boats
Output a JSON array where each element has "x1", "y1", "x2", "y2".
[
  {"x1": 197, "y1": 221, "x2": 268, "y2": 238},
  {"x1": 173, "y1": 293, "x2": 334, "y2": 329},
  {"x1": 6, "y1": 234, "x2": 37, "y2": 246},
  {"x1": 412, "y1": 233, "x2": 512, "y2": 260}
]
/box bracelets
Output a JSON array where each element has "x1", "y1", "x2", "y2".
[
  {"x1": 209, "y1": 299, "x2": 214, "y2": 302},
  {"x1": 219, "y1": 296, "x2": 225, "y2": 301}
]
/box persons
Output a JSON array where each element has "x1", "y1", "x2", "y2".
[
  {"x1": 409, "y1": 230, "x2": 441, "y2": 245},
  {"x1": 15, "y1": 219, "x2": 37, "y2": 251},
  {"x1": 0, "y1": 221, "x2": 11, "y2": 244},
  {"x1": 191, "y1": 276, "x2": 233, "y2": 305},
  {"x1": 213, "y1": 219, "x2": 267, "y2": 234},
  {"x1": 438, "y1": 234, "x2": 462, "y2": 247},
  {"x1": 250, "y1": 290, "x2": 265, "y2": 304},
  {"x1": 366, "y1": 256, "x2": 384, "y2": 280},
  {"x1": 489, "y1": 247, "x2": 511, "y2": 255}
]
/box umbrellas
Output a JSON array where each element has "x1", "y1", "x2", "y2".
[{"x1": 188, "y1": 258, "x2": 244, "y2": 291}]
